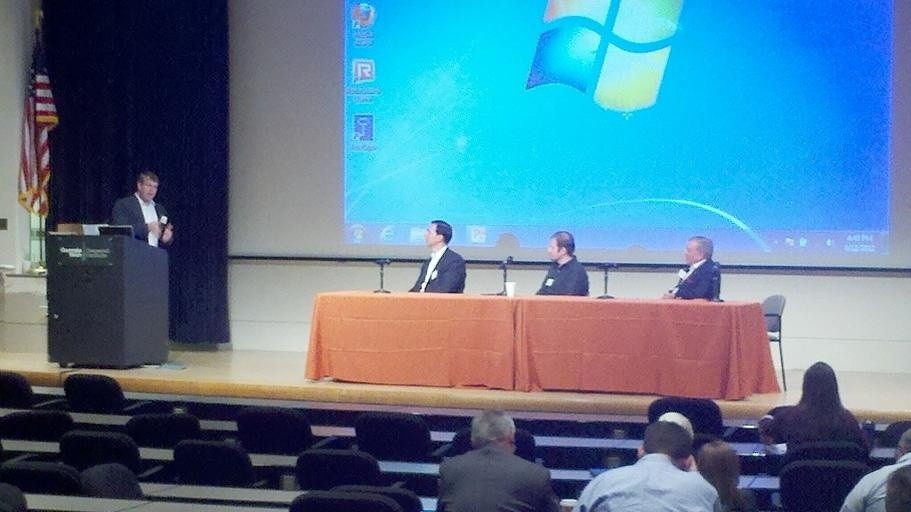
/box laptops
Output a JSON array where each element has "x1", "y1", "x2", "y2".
[{"x1": 98, "y1": 225, "x2": 135, "y2": 236}]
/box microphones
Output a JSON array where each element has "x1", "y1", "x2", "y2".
[
  {"x1": 376, "y1": 257, "x2": 391, "y2": 266},
  {"x1": 159, "y1": 214, "x2": 168, "y2": 233},
  {"x1": 598, "y1": 264, "x2": 618, "y2": 269},
  {"x1": 500, "y1": 256, "x2": 513, "y2": 269}
]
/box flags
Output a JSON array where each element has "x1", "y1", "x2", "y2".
[{"x1": 17, "y1": 41, "x2": 60, "y2": 218}]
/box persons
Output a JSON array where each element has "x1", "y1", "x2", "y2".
[
  {"x1": 408, "y1": 220, "x2": 465, "y2": 293},
  {"x1": 573, "y1": 412, "x2": 761, "y2": 511},
  {"x1": 661, "y1": 237, "x2": 720, "y2": 299},
  {"x1": 437, "y1": 409, "x2": 560, "y2": 512},
  {"x1": 761, "y1": 362, "x2": 911, "y2": 511},
  {"x1": 108, "y1": 171, "x2": 178, "y2": 248},
  {"x1": 535, "y1": 231, "x2": 589, "y2": 295}
]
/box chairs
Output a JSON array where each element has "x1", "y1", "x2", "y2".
[{"x1": 757, "y1": 293, "x2": 792, "y2": 394}]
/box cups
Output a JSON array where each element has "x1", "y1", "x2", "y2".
[{"x1": 504, "y1": 282, "x2": 517, "y2": 298}]
